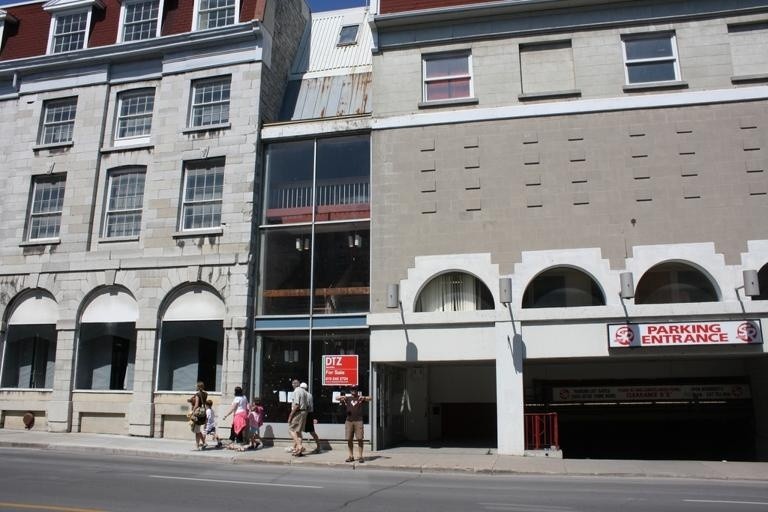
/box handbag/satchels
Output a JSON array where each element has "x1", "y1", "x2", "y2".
[{"x1": 191, "y1": 407, "x2": 206, "y2": 425}]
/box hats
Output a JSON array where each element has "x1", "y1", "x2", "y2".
[
  {"x1": 300, "y1": 383, "x2": 307, "y2": 388},
  {"x1": 350, "y1": 384, "x2": 357, "y2": 388}
]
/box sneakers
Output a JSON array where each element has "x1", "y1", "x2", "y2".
[
  {"x1": 312, "y1": 449, "x2": 320, "y2": 454},
  {"x1": 255, "y1": 443, "x2": 260, "y2": 448},
  {"x1": 346, "y1": 456, "x2": 354, "y2": 462},
  {"x1": 359, "y1": 458, "x2": 363, "y2": 462},
  {"x1": 248, "y1": 446, "x2": 254, "y2": 449}
]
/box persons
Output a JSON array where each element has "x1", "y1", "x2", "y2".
[
  {"x1": 284, "y1": 383, "x2": 322, "y2": 453},
  {"x1": 222, "y1": 386, "x2": 253, "y2": 452},
  {"x1": 189, "y1": 381, "x2": 209, "y2": 451},
  {"x1": 247, "y1": 406, "x2": 264, "y2": 450},
  {"x1": 197, "y1": 399, "x2": 223, "y2": 449},
  {"x1": 250, "y1": 397, "x2": 263, "y2": 448},
  {"x1": 336, "y1": 384, "x2": 374, "y2": 463},
  {"x1": 288, "y1": 379, "x2": 308, "y2": 457}
]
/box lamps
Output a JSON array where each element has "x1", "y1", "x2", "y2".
[
  {"x1": 735, "y1": 270, "x2": 761, "y2": 298},
  {"x1": 617, "y1": 272, "x2": 635, "y2": 323},
  {"x1": 499, "y1": 277, "x2": 519, "y2": 338},
  {"x1": 385, "y1": 282, "x2": 410, "y2": 345}
]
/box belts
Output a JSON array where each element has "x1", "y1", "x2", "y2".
[{"x1": 296, "y1": 409, "x2": 305, "y2": 411}]
